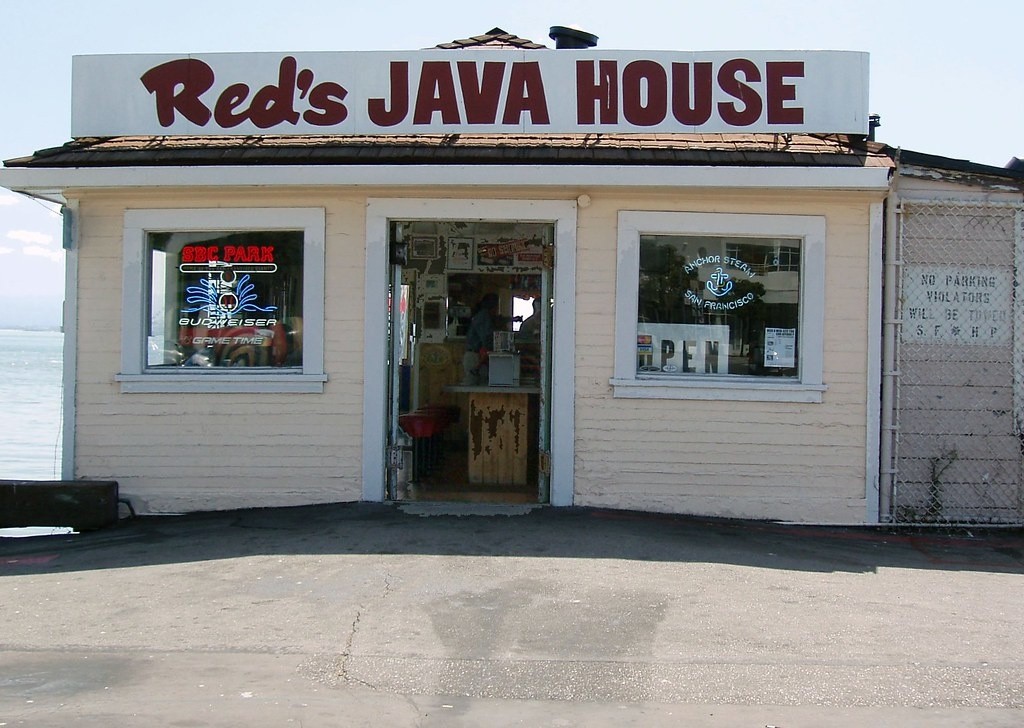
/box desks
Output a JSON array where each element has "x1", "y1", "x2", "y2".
[{"x1": 441, "y1": 377, "x2": 542, "y2": 486}]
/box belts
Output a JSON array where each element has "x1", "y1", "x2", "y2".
[{"x1": 468, "y1": 348, "x2": 480, "y2": 352}]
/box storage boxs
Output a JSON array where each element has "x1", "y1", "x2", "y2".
[{"x1": 489, "y1": 351, "x2": 520, "y2": 387}]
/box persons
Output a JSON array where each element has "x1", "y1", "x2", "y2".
[
  {"x1": 518, "y1": 297, "x2": 541, "y2": 332},
  {"x1": 462, "y1": 293, "x2": 499, "y2": 377}
]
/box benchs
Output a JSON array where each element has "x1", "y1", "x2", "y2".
[{"x1": 399, "y1": 406, "x2": 460, "y2": 482}]
